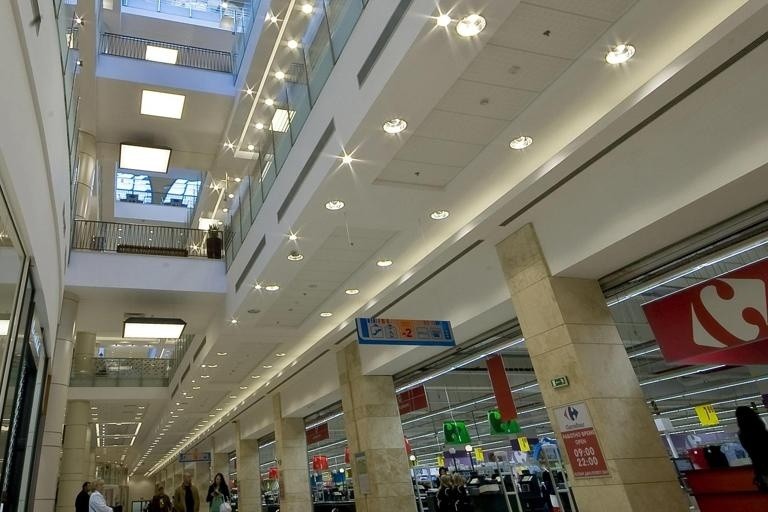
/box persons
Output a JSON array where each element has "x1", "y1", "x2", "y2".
[
  {"x1": 75, "y1": 478, "x2": 113, "y2": 512},
  {"x1": 436, "y1": 467, "x2": 468, "y2": 512},
  {"x1": 206, "y1": 472, "x2": 230, "y2": 512},
  {"x1": 174, "y1": 472, "x2": 199, "y2": 512},
  {"x1": 143, "y1": 487, "x2": 174, "y2": 512},
  {"x1": 735, "y1": 406, "x2": 768, "y2": 489}
]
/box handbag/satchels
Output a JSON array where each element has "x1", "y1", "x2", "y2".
[{"x1": 219, "y1": 502, "x2": 232, "y2": 512}]
